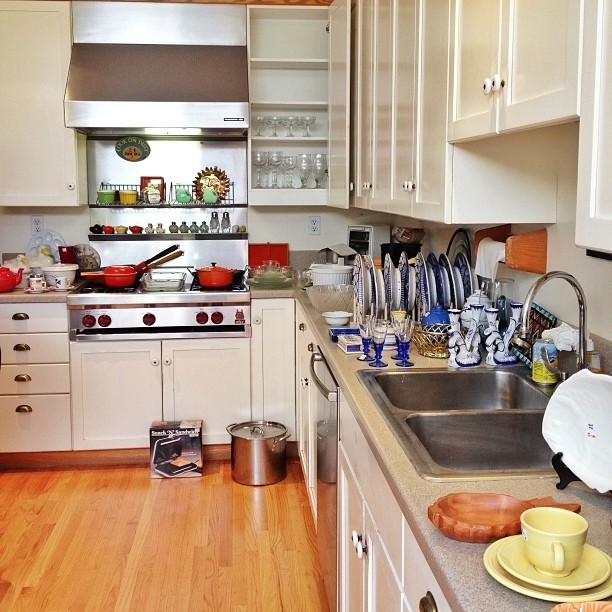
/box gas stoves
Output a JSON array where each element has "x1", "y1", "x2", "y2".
[
  {"x1": 191, "y1": 276, "x2": 242, "y2": 291},
  {"x1": 79, "y1": 282, "x2": 141, "y2": 292}
]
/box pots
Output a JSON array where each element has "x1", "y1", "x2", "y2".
[
  {"x1": 79, "y1": 263, "x2": 138, "y2": 288},
  {"x1": 97, "y1": 242, "x2": 181, "y2": 272},
  {"x1": 82, "y1": 250, "x2": 186, "y2": 287},
  {"x1": 187, "y1": 265, "x2": 250, "y2": 283},
  {"x1": 197, "y1": 262, "x2": 237, "y2": 287},
  {"x1": 225, "y1": 418, "x2": 292, "y2": 486}
]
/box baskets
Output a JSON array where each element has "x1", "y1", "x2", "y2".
[{"x1": 414, "y1": 323, "x2": 451, "y2": 359}]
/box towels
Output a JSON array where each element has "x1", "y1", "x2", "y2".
[{"x1": 542, "y1": 323, "x2": 595, "y2": 354}]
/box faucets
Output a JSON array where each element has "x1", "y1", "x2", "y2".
[{"x1": 518, "y1": 271, "x2": 587, "y2": 372}]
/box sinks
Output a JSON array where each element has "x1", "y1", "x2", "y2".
[
  {"x1": 404, "y1": 412, "x2": 554, "y2": 471},
  {"x1": 375, "y1": 370, "x2": 550, "y2": 410}
]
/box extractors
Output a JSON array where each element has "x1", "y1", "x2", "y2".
[{"x1": 57, "y1": 1, "x2": 252, "y2": 144}]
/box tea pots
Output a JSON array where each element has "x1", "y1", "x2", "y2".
[
  {"x1": 0, "y1": 265, "x2": 25, "y2": 293},
  {"x1": 116, "y1": 225, "x2": 128, "y2": 234},
  {"x1": 102, "y1": 225, "x2": 114, "y2": 234},
  {"x1": 89, "y1": 224, "x2": 102, "y2": 234},
  {"x1": 129, "y1": 226, "x2": 144, "y2": 234}
]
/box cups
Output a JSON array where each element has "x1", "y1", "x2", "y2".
[
  {"x1": 118, "y1": 190, "x2": 139, "y2": 205},
  {"x1": 56, "y1": 276, "x2": 71, "y2": 288},
  {"x1": 497, "y1": 279, "x2": 515, "y2": 328},
  {"x1": 29, "y1": 275, "x2": 46, "y2": 289},
  {"x1": 96, "y1": 189, "x2": 116, "y2": 205},
  {"x1": 294, "y1": 268, "x2": 310, "y2": 288},
  {"x1": 281, "y1": 265, "x2": 294, "y2": 279},
  {"x1": 262, "y1": 259, "x2": 280, "y2": 272},
  {"x1": 519, "y1": 508, "x2": 588, "y2": 579},
  {"x1": 56, "y1": 244, "x2": 78, "y2": 263}
]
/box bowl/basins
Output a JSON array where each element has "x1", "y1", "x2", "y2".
[
  {"x1": 322, "y1": 310, "x2": 352, "y2": 326},
  {"x1": 41, "y1": 262, "x2": 79, "y2": 286},
  {"x1": 305, "y1": 284, "x2": 353, "y2": 315},
  {"x1": 311, "y1": 264, "x2": 353, "y2": 285},
  {"x1": 394, "y1": 228, "x2": 426, "y2": 242}
]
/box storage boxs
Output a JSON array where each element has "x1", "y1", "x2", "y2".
[{"x1": 150, "y1": 420, "x2": 203, "y2": 479}]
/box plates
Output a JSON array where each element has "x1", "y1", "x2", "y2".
[
  {"x1": 53, "y1": 286, "x2": 74, "y2": 291},
  {"x1": 484, "y1": 535, "x2": 612, "y2": 604},
  {"x1": 439, "y1": 253, "x2": 464, "y2": 311},
  {"x1": 427, "y1": 252, "x2": 451, "y2": 310},
  {"x1": 446, "y1": 228, "x2": 474, "y2": 265},
  {"x1": 398, "y1": 251, "x2": 416, "y2": 311},
  {"x1": 498, "y1": 535, "x2": 610, "y2": 591},
  {"x1": 353, "y1": 253, "x2": 373, "y2": 322},
  {"x1": 415, "y1": 252, "x2": 437, "y2": 312},
  {"x1": 454, "y1": 252, "x2": 479, "y2": 302},
  {"x1": 26, "y1": 288, "x2": 50, "y2": 293},
  {"x1": 364, "y1": 253, "x2": 385, "y2": 321},
  {"x1": 384, "y1": 253, "x2": 401, "y2": 317},
  {"x1": 542, "y1": 368, "x2": 612, "y2": 494}
]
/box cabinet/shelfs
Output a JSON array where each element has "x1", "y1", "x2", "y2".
[
  {"x1": 250, "y1": 298, "x2": 296, "y2": 442},
  {"x1": 403, "y1": 519, "x2": 451, "y2": 611},
  {"x1": 576, "y1": 1, "x2": 611, "y2": 255},
  {"x1": 392, "y1": 1, "x2": 556, "y2": 224},
  {"x1": 342, "y1": 467, "x2": 404, "y2": 611},
  {"x1": 246, "y1": 1, "x2": 351, "y2": 210},
  {"x1": 70, "y1": 341, "x2": 250, "y2": 451},
  {"x1": 1, "y1": 1, "x2": 87, "y2": 206},
  {"x1": 448, "y1": 1, "x2": 583, "y2": 144},
  {"x1": 295, "y1": 299, "x2": 317, "y2": 489},
  {"x1": 0, "y1": 302, "x2": 73, "y2": 453},
  {"x1": 353, "y1": 0, "x2": 391, "y2": 213},
  {"x1": 89, "y1": 182, "x2": 247, "y2": 240}
]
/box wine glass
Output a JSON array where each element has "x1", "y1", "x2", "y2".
[
  {"x1": 252, "y1": 151, "x2": 267, "y2": 188},
  {"x1": 394, "y1": 319, "x2": 414, "y2": 367},
  {"x1": 265, "y1": 116, "x2": 282, "y2": 136},
  {"x1": 392, "y1": 312, "x2": 407, "y2": 352},
  {"x1": 298, "y1": 155, "x2": 311, "y2": 188},
  {"x1": 369, "y1": 323, "x2": 387, "y2": 365},
  {"x1": 282, "y1": 115, "x2": 299, "y2": 137},
  {"x1": 311, "y1": 154, "x2": 326, "y2": 188},
  {"x1": 299, "y1": 116, "x2": 316, "y2": 137},
  {"x1": 283, "y1": 156, "x2": 298, "y2": 189},
  {"x1": 357, "y1": 314, "x2": 375, "y2": 361},
  {"x1": 267, "y1": 151, "x2": 283, "y2": 189},
  {"x1": 252, "y1": 115, "x2": 265, "y2": 136},
  {"x1": 393, "y1": 315, "x2": 411, "y2": 359}
]
